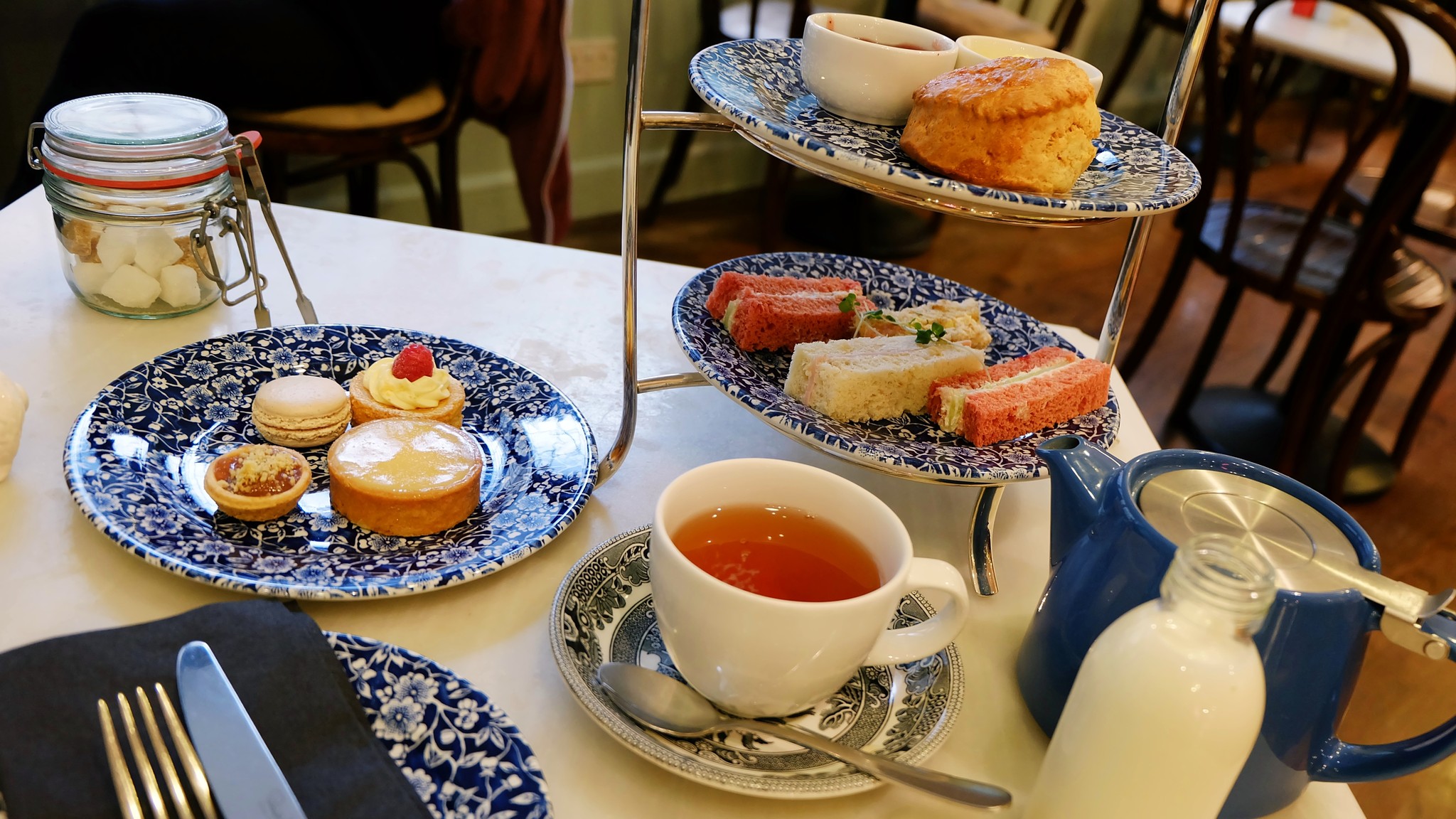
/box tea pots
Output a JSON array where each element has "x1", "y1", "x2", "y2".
[{"x1": 1016, "y1": 435, "x2": 1456, "y2": 819}]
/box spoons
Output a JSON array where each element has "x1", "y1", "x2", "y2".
[{"x1": 596, "y1": 662, "x2": 1012, "y2": 808}]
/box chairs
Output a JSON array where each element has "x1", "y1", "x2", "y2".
[
  {"x1": 642, "y1": 0, "x2": 945, "y2": 259},
  {"x1": 245, "y1": 40, "x2": 480, "y2": 234},
  {"x1": 1119, "y1": 0, "x2": 1456, "y2": 504}
]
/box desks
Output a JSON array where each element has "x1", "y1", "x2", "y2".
[
  {"x1": 1110, "y1": 1, "x2": 1456, "y2": 228},
  {"x1": 0, "y1": 178, "x2": 1367, "y2": 819}
]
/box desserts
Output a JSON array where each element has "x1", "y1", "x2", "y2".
[
  {"x1": 705, "y1": 271, "x2": 1110, "y2": 444},
  {"x1": 203, "y1": 444, "x2": 311, "y2": 521},
  {"x1": 349, "y1": 343, "x2": 465, "y2": 431},
  {"x1": 327, "y1": 416, "x2": 484, "y2": 537},
  {"x1": 899, "y1": 56, "x2": 1104, "y2": 196},
  {"x1": 251, "y1": 375, "x2": 354, "y2": 446}
]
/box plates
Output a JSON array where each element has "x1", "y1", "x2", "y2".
[
  {"x1": 61, "y1": 322, "x2": 600, "y2": 602},
  {"x1": 546, "y1": 524, "x2": 968, "y2": 801},
  {"x1": 321, "y1": 630, "x2": 554, "y2": 819}
]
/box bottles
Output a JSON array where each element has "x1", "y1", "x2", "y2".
[
  {"x1": 27, "y1": 92, "x2": 262, "y2": 319},
  {"x1": 1018, "y1": 532, "x2": 1281, "y2": 819}
]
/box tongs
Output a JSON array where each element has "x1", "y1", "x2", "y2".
[{"x1": 224, "y1": 135, "x2": 320, "y2": 328}]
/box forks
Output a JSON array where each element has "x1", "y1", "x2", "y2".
[{"x1": 98, "y1": 681, "x2": 219, "y2": 818}]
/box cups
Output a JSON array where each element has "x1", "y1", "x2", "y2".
[
  {"x1": 957, "y1": 35, "x2": 1104, "y2": 102},
  {"x1": 648, "y1": 458, "x2": 970, "y2": 717},
  {"x1": 801, "y1": 13, "x2": 958, "y2": 127}
]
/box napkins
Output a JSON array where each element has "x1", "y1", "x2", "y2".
[{"x1": 1, "y1": 603, "x2": 434, "y2": 819}]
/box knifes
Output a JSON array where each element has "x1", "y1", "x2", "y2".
[{"x1": 176, "y1": 641, "x2": 308, "y2": 818}]
